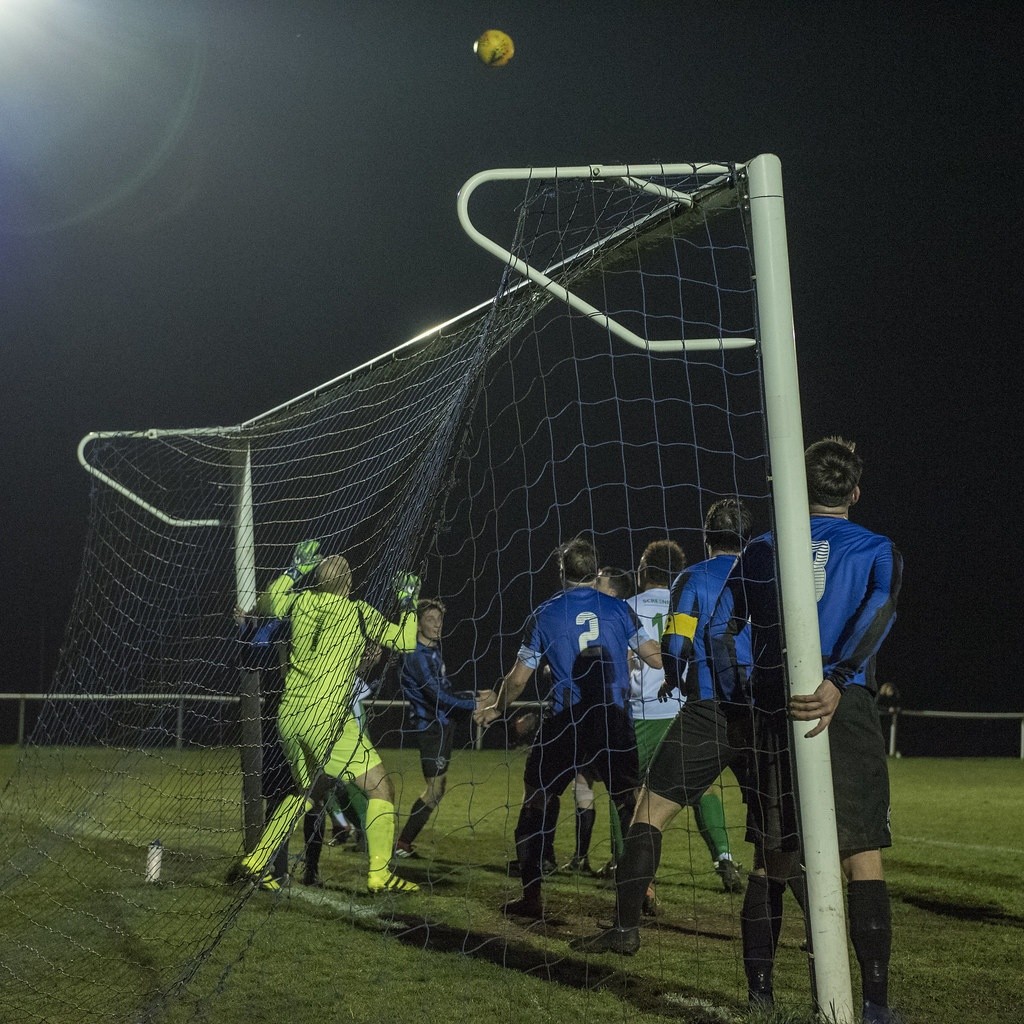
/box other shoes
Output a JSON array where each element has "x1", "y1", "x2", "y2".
[
  {"x1": 862, "y1": 1000, "x2": 900, "y2": 1024},
  {"x1": 749, "y1": 990, "x2": 774, "y2": 1011}
]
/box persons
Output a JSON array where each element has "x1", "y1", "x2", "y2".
[{"x1": 220, "y1": 434, "x2": 906, "y2": 1024}]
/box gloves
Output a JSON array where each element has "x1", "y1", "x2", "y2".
[
  {"x1": 284, "y1": 537, "x2": 323, "y2": 585},
  {"x1": 392, "y1": 570, "x2": 421, "y2": 613}
]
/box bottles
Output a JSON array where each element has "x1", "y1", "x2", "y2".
[{"x1": 146, "y1": 839, "x2": 162, "y2": 882}]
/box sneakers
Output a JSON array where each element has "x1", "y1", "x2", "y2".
[
  {"x1": 596, "y1": 861, "x2": 616, "y2": 877},
  {"x1": 393, "y1": 844, "x2": 425, "y2": 861},
  {"x1": 506, "y1": 851, "x2": 557, "y2": 875},
  {"x1": 643, "y1": 888, "x2": 665, "y2": 916},
  {"x1": 368, "y1": 871, "x2": 420, "y2": 894},
  {"x1": 721, "y1": 861, "x2": 745, "y2": 894},
  {"x1": 228, "y1": 860, "x2": 282, "y2": 892},
  {"x1": 343, "y1": 836, "x2": 369, "y2": 853},
  {"x1": 500, "y1": 895, "x2": 543, "y2": 918},
  {"x1": 570, "y1": 926, "x2": 641, "y2": 956},
  {"x1": 559, "y1": 854, "x2": 592, "y2": 878},
  {"x1": 329, "y1": 823, "x2": 355, "y2": 847}
]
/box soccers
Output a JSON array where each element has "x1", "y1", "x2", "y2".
[{"x1": 473, "y1": 29, "x2": 515, "y2": 69}]
